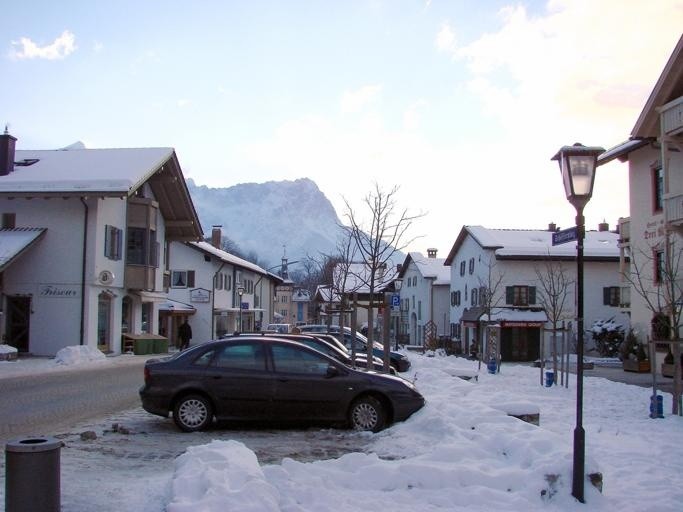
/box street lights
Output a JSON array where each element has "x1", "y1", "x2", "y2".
[
  {"x1": 237, "y1": 285, "x2": 244, "y2": 334},
  {"x1": 551, "y1": 142, "x2": 606, "y2": 502}
]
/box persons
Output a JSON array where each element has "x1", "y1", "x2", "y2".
[{"x1": 177, "y1": 319, "x2": 192, "y2": 352}]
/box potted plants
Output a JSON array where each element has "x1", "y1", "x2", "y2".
[
  {"x1": 662, "y1": 349, "x2": 683, "y2": 379},
  {"x1": 620, "y1": 327, "x2": 650, "y2": 373}
]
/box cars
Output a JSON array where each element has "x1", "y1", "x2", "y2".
[{"x1": 139, "y1": 324, "x2": 425, "y2": 433}]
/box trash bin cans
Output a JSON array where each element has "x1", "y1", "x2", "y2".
[{"x1": 5, "y1": 437, "x2": 65, "y2": 512}]
